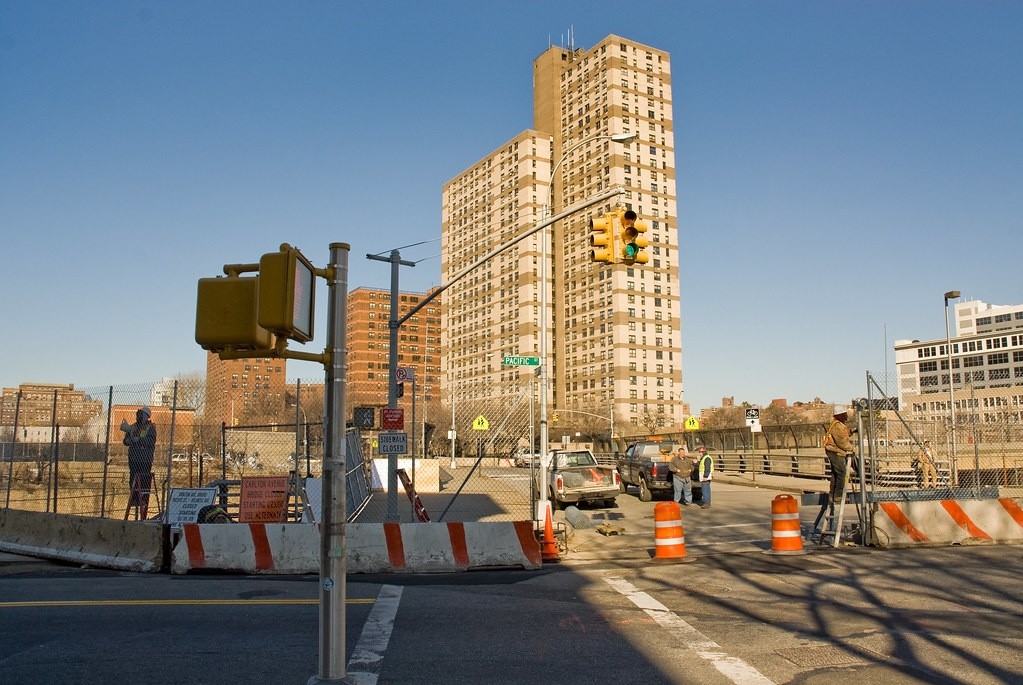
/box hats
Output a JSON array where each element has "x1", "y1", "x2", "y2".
[{"x1": 924, "y1": 439, "x2": 930, "y2": 442}]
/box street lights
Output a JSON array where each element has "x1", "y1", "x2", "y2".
[
  {"x1": 534, "y1": 131, "x2": 638, "y2": 536},
  {"x1": 224, "y1": 391, "x2": 242, "y2": 431},
  {"x1": 291, "y1": 404, "x2": 307, "y2": 456},
  {"x1": 944, "y1": 289, "x2": 961, "y2": 486}
]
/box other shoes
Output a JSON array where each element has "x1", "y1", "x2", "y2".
[
  {"x1": 829, "y1": 496, "x2": 849, "y2": 503},
  {"x1": 697, "y1": 501, "x2": 711, "y2": 508}
]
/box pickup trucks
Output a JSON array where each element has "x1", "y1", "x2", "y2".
[
  {"x1": 534, "y1": 449, "x2": 621, "y2": 514},
  {"x1": 614, "y1": 440, "x2": 700, "y2": 502}
]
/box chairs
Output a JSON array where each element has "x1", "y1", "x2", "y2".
[{"x1": 578, "y1": 458, "x2": 589, "y2": 464}]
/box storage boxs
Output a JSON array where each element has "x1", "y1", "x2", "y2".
[{"x1": 645, "y1": 455, "x2": 674, "y2": 462}]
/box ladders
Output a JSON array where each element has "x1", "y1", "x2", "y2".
[
  {"x1": 278, "y1": 470, "x2": 316, "y2": 524},
  {"x1": 123, "y1": 472, "x2": 162, "y2": 523},
  {"x1": 819, "y1": 454, "x2": 853, "y2": 548},
  {"x1": 397, "y1": 468, "x2": 432, "y2": 523}
]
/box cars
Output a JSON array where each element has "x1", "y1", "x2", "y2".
[
  {"x1": 690, "y1": 445, "x2": 724, "y2": 460},
  {"x1": 166, "y1": 451, "x2": 214, "y2": 467}
]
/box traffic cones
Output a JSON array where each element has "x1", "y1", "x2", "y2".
[{"x1": 540, "y1": 505, "x2": 561, "y2": 563}]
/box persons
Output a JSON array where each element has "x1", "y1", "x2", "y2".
[
  {"x1": 918, "y1": 439, "x2": 939, "y2": 488},
  {"x1": 693, "y1": 446, "x2": 714, "y2": 509},
  {"x1": 823, "y1": 405, "x2": 858, "y2": 504},
  {"x1": 668, "y1": 448, "x2": 695, "y2": 507},
  {"x1": 120, "y1": 407, "x2": 157, "y2": 506}
]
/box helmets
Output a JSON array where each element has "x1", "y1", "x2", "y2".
[
  {"x1": 141, "y1": 407, "x2": 151, "y2": 418},
  {"x1": 833, "y1": 406, "x2": 848, "y2": 417},
  {"x1": 698, "y1": 447, "x2": 706, "y2": 451}
]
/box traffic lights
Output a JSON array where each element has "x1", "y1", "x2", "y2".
[
  {"x1": 589, "y1": 211, "x2": 615, "y2": 265},
  {"x1": 553, "y1": 414, "x2": 559, "y2": 422},
  {"x1": 256, "y1": 245, "x2": 317, "y2": 343},
  {"x1": 634, "y1": 220, "x2": 649, "y2": 264},
  {"x1": 618, "y1": 211, "x2": 639, "y2": 265}
]
